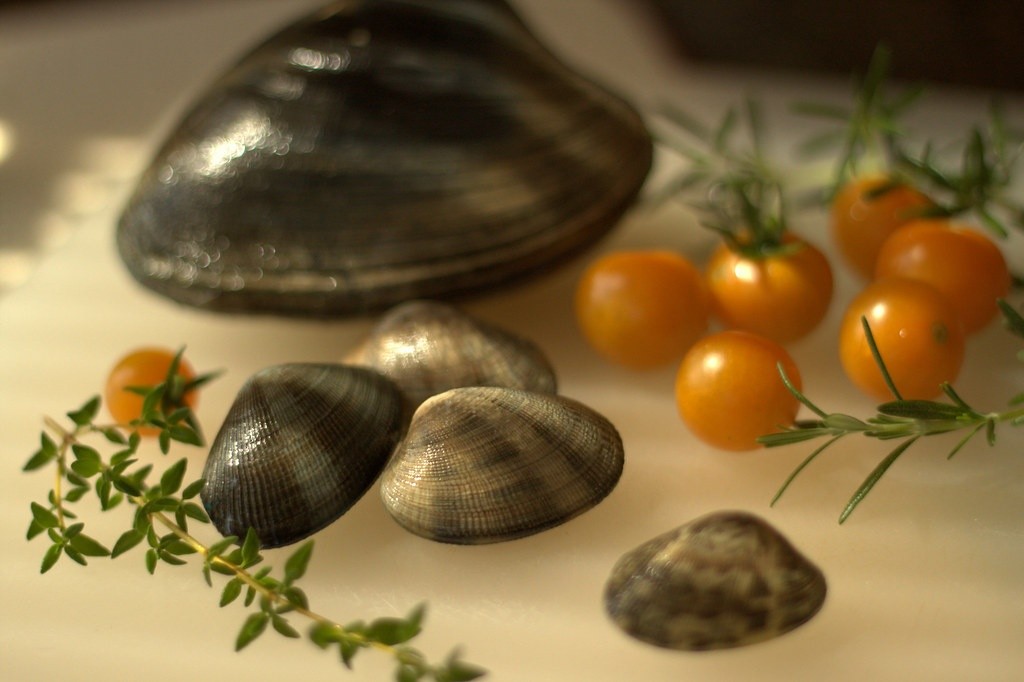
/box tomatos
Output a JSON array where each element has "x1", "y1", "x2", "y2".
[
  {"x1": 103, "y1": 347, "x2": 202, "y2": 440},
  {"x1": 575, "y1": 169, "x2": 1012, "y2": 453}
]
